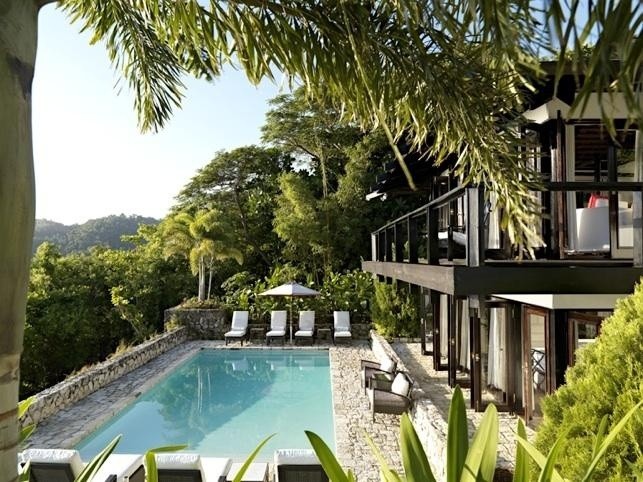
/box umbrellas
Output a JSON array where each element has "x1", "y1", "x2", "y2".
[{"x1": 255, "y1": 279, "x2": 322, "y2": 346}]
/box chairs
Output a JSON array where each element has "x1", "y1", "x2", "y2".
[
  {"x1": 146, "y1": 452, "x2": 233, "y2": 481},
  {"x1": 275, "y1": 449, "x2": 327, "y2": 480},
  {"x1": 224, "y1": 309, "x2": 353, "y2": 347},
  {"x1": 21, "y1": 450, "x2": 144, "y2": 482},
  {"x1": 360, "y1": 355, "x2": 414, "y2": 424},
  {"x1": 425, "y1": 197, "x2": 493, "y2": 260}
]
